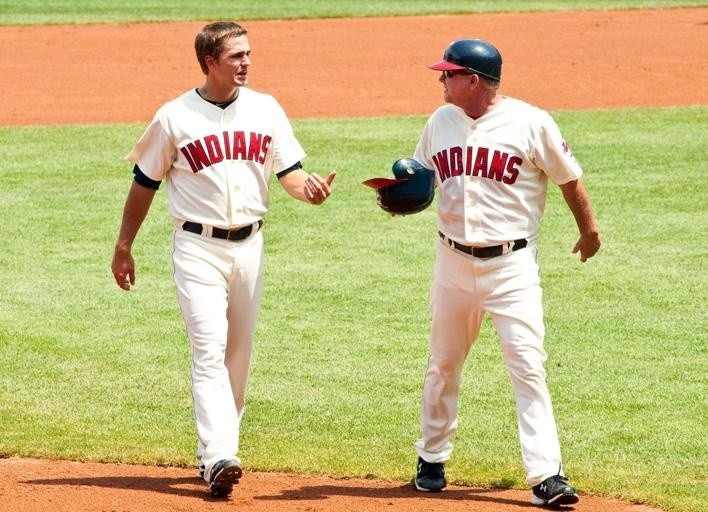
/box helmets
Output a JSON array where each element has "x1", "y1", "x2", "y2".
[
  {"x1": 361, "y1": 157, "x2": 436, "y2": 215},
  {"x1": 426, "y1": 39, "x2": 502, "y2": 83}
]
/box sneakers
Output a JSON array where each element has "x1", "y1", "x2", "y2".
[
  {"x1": 210, "y1": 458, "x2": 243, "y2": 497},
  {"x1": 414, "y1": 454, "x2": 448, "y2": 492},
  {"x1": 530, "y1": 474, "x2": 579, "y2": 507}
]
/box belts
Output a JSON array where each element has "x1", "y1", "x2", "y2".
[
  {"x1": 438, "y1": 229, "x2": 528, "y2": 259},
  {"x1": 182, "y1": 219, "x2": 263, "y2": 241}
]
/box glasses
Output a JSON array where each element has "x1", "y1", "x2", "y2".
[{"x1": 443, "y1": 70, "x2": 472, "y2": 78}]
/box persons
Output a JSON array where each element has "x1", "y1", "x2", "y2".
[
  {"x1": 112, "y1": 21, "x2": 336, "y2": 497},
  {"x1": 377, "y1": 38, "x2": 600, "y2": 508}
]
